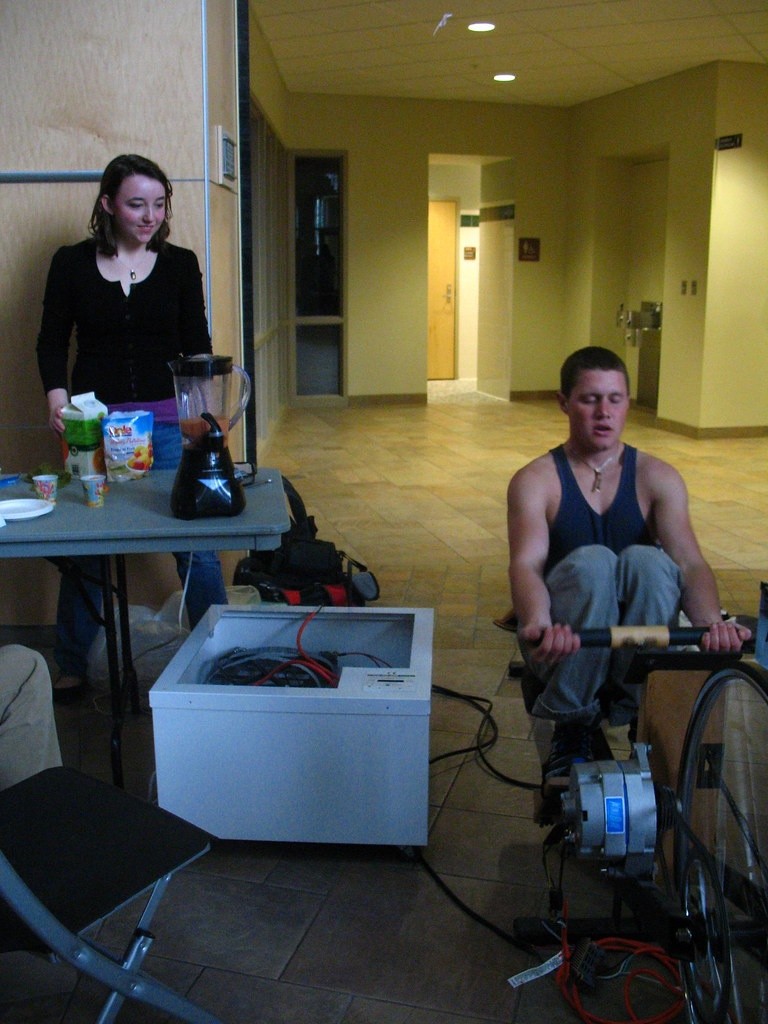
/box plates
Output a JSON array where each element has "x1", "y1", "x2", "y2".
[{"x1": 0, "y1": 498, "x2": 53, "y2": 521}]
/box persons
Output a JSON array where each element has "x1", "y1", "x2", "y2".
[
  {"x1": 36, "y1": 153, "x2": 229, "y2": 701},
  {"x1": 507, "y1": 346, "x2": 753, "y2": 787}
]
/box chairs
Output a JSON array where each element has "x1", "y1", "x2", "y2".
[{"x1": 0, "y1": 765, "x2": 226, "y2": 1024}]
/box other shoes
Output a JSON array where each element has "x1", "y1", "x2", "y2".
[{"x1": 53, "y1": 675, "x2": 88, "y2": 703}]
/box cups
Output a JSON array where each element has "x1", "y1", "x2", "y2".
[
  {"x1": 80, "y1": 474, "x2": 106, "y2": 507},
  {"x1": 32, "y1": 474, "x2": 58, "y2": 505}
]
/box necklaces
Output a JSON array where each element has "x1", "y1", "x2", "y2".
[
  {"x1": 568, "y1": 446, "x2": 613, "y2": 493},
  {"x1": 118, "y1": 258, "x2": 142, "y2": 280}
]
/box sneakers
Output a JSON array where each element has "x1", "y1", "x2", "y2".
[{"x1": 543, "y1": 723, "x2": 594, "y2": 785}]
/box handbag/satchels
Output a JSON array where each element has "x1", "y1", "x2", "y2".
[
  {"x1": 251, "y1": 475, "x2": 367, "y2": 586},
  {"x1": 233, "y1": 556, "x2": 379, "y2": 607}
]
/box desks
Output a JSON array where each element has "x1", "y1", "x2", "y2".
[{"x1": 0, "y1": 465, "x2": 292, "y2": 794}]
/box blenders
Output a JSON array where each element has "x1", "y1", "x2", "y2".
[{"x1": 167, "y1": 354, "x2": 252, "y2": 520}]
